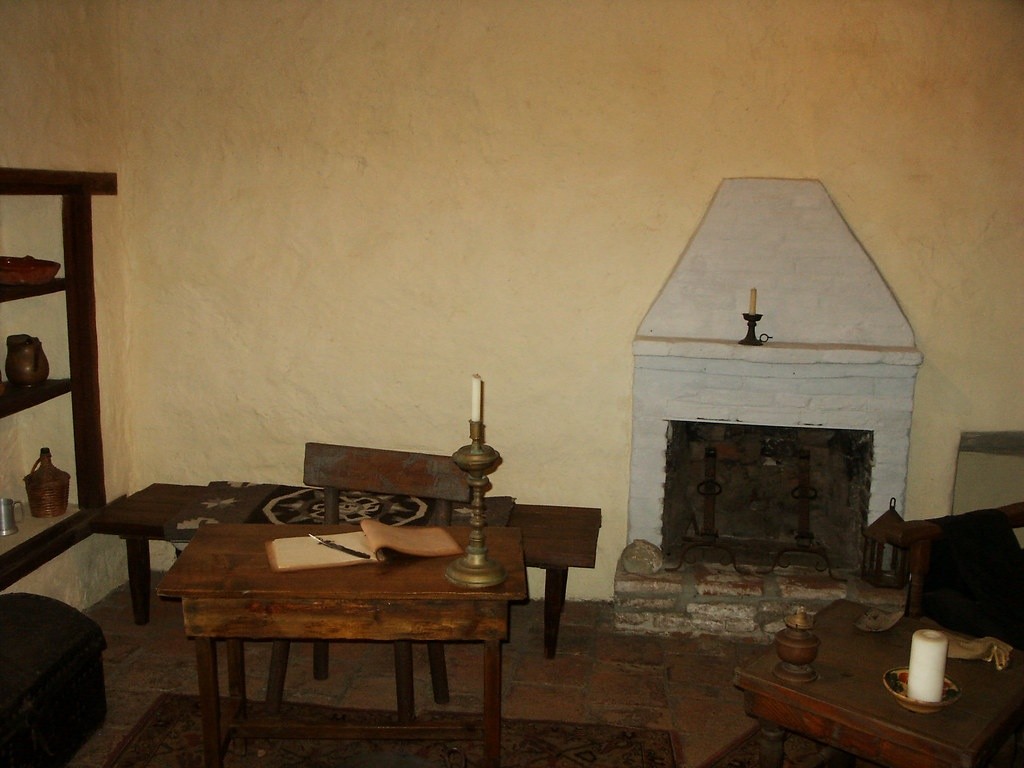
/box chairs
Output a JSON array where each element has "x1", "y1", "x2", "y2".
[
  {"x1": 262, "y1": 442, "x2": 470, "y2": 726},
  {"x1": 885, "y1": 503, "x2": 1024, "y2": 652}
]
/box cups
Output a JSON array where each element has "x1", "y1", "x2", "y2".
[{"x1": 0, "y1": 498, "x2": 24, "y2": 536}]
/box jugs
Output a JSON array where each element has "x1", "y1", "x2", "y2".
[{"x1": 5, "y1": 335, "x2": 49, "y2": 387}]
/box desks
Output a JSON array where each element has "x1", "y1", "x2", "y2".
[
  {"x1": 154, "y1": 524, "x2": 529, "y2": 768},
  {"x1": 732, "y1": 599, "x2": 1024, "y2": 768}
]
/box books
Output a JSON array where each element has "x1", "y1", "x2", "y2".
[{"x1": 264, "y1": 519, "x2": 463, "y2": 572}]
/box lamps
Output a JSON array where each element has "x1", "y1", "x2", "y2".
[{"x1": 862, "y1": 498, "x2": 910, "y2": 590}]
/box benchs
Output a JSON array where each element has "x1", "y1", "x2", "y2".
[{"x1": 91, "y1": 483, "x2": 603, "y2": 660}]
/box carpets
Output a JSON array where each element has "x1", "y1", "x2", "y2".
[
  {"x1": 102, "y1": 693, "x2": 686, "y2": 768},
  {"x1": 695, "y1": 724, "x2": 1024, "y2": 768}
]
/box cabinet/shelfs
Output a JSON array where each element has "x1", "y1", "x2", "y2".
[{"x1": 0, "y1": 165, "x2": 129, "y2": 590}]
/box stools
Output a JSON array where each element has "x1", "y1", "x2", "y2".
[{"x1": 0, "y1": 592, "x2": 105, "y2": 768}]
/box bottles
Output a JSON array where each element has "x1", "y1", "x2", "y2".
[{"x1": 24, "y1": 447, "x2": 71, "y2": 518}]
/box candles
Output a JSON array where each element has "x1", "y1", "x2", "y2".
[
  {"x1": 907, "y1": 630, "x2": 949, "y2": 702},
  {"x1": 748, "y1": 286, "x2": 757, "y2": 316},
  {"x1": 471, "y1": 374, "x2": 481, "y2": 422}
]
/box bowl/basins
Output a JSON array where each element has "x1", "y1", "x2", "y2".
[
  {"x1": 0, "y1": 255, "x2": 61, "y2": 286},
  {"x1": 882, "y1": 666, "x2": 962, "y2": 715}
]
